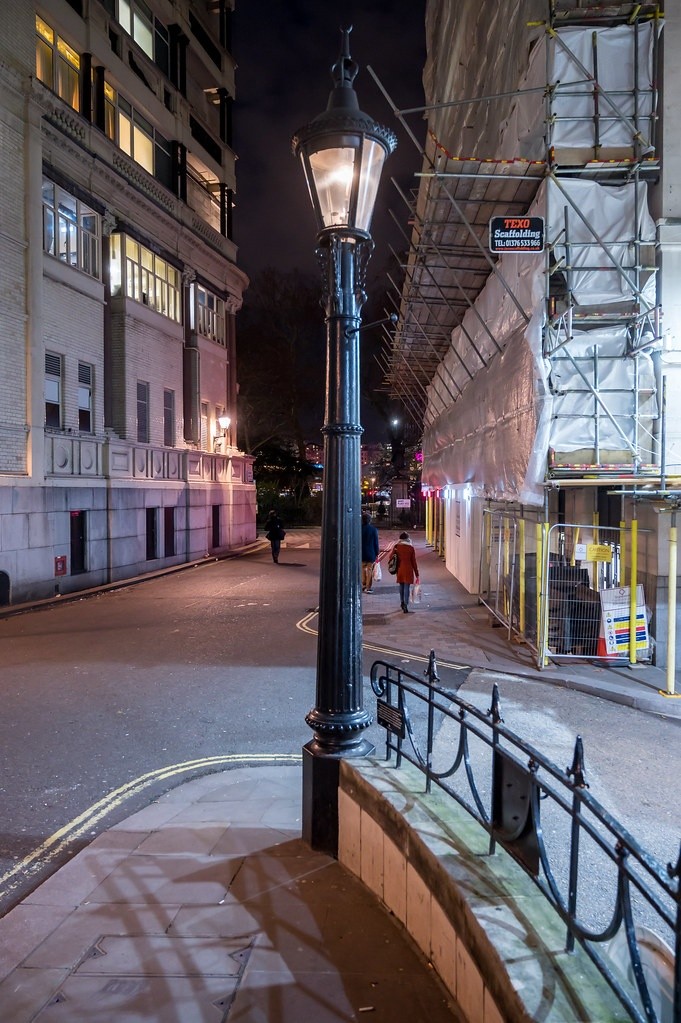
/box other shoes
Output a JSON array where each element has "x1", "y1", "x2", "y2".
[
  {"x1": 366, "y1": 589, "x2": 373, "y2": 593},
  {"x1": 403, "y1": 605, "x2": 408, "y2": 613},
  {"x1": 272, "y1": 557, "x2": 278, "y2": 563},
  {"x1": 362, "y1": 585, "x2": 366, "y2": 591},
  {"x1": 401, "y1": 602, "x2": 404, "y2": 609}
]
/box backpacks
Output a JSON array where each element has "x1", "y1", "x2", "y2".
[{"x1": 266, "y1": 518, "x2": 286, "y2": 540}]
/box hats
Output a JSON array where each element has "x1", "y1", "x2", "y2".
[{"x1": 400, "y1": 532, "x2": 410, "y2": 539}]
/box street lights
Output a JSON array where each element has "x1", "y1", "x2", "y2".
[{"x1": 291, "y1": 22, "x2": 403, "y2": 860}]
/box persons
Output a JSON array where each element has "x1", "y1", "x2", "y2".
[
  {"x1": 390, "y1": 530, "x2": 419, "y2": 612},
  {"x1": 263, "y1": 511, "x2": 286, "y2": 563},
  {"x1": 361, "y1": 513, "x2": 382, "y2": 593}
]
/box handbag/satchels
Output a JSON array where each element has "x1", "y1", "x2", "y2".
[
  {"x1": 373, "y1": 556, "x2": 382, "y2": 581},
  {"x1": 389, "y1": 547, "x2": 398, "y2": 574},
  {"x1": 410, "y1": 576, "x2": 421, "y2": 604}
]
